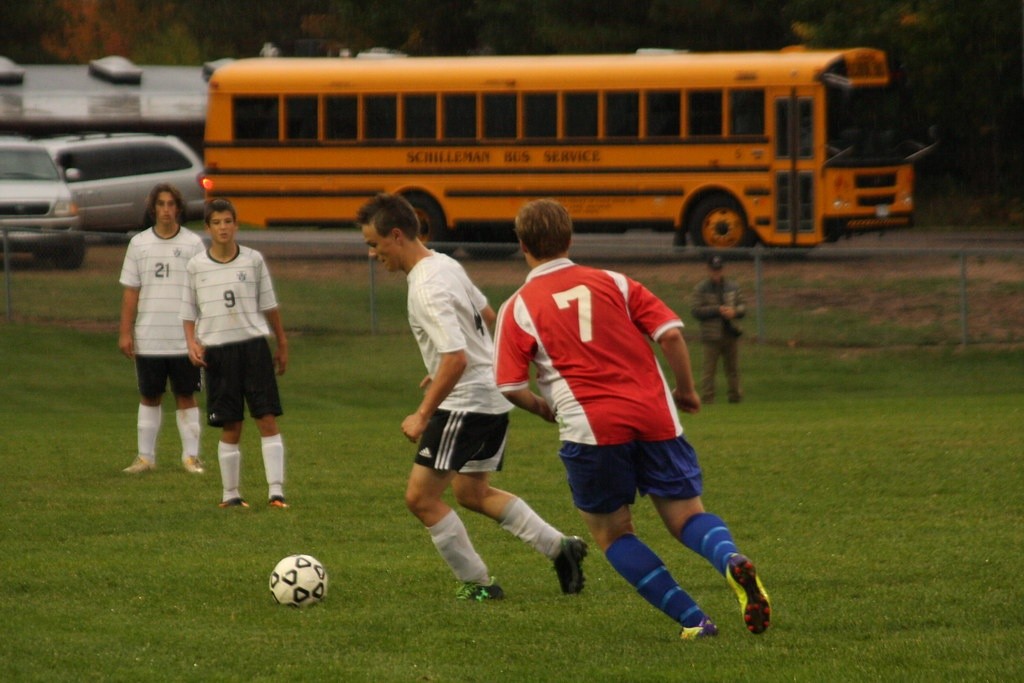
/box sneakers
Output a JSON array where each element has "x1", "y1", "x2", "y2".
[
  {"x1": 181, "y1": 457, "x2": 205, "y2": 474},
  {"x1": 454, "y1": 576, "x2": 505, "y2": 602},
  {"x1": 679, "y1": 615, "x2": 719, "y2": 642},
  {"x1": 123, "y1": 459, "x2": 157, "y2": 477},
  {"x1": 551, "y1": 535, "x2": 589, "y2": 595},
  {"x1": 726, "y1": 553, "x2": 771, "y2": 635},
  {"x1": 268, "y1": 495, "x2": 288, "y2": 508}
]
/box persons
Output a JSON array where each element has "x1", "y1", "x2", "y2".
[
  {"x1": 690, "y1": 256, "x2": 748, "y2": 405},
  {"x1": 178, "y1": 197, "x2": 292, "y2": 509},
  {"x1": 494, "y1": 201, "x2": 772, "y2": 640},
  {"x1": 355, "y1": 191, "x2": 588, "y2": 602},
  {"x1": 117, "y1": 184, "x2": 207, "y2": 476}
]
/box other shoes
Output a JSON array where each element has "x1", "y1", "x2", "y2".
[
  {"x1": 728, "y1": 392, "x2": 743, "y2": 403},
  {"x1": 702, "y1": 394, "x2": 715, "y2": 404}
]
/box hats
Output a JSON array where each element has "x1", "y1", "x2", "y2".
[{"x1": 707, "y1": 256, "x2": 724, "y2": 271}]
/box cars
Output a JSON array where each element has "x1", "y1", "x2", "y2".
[{"x1": 0, "y1": 137, "x2": 83, "y2": 266}]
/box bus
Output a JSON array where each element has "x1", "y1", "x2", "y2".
[{"x1": 197, "y1": 51, "x2": 913, "y2": 257}]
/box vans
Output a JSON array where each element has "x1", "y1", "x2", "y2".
[{"x1": 38, "y1": 134, "x2": 207, "y2": 231}]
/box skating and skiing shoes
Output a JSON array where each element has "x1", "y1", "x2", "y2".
[{"x1": 218, "y1": 498, "x2": 253, "y2": 510}]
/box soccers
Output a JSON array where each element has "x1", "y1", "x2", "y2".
[{"x1": 268, "y1": 553, "x2": 328, "y2": 608}]
